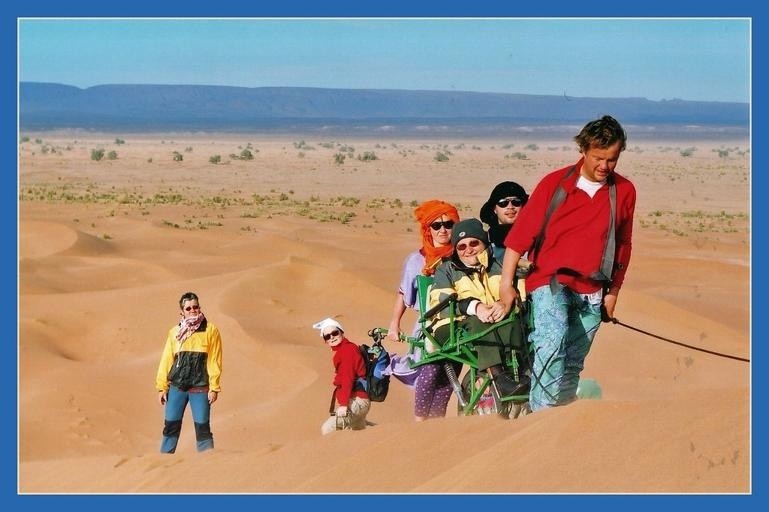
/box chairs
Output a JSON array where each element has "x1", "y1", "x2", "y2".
[{"x1": 367, "y1": 275, "x2": 532, "y2": 414}]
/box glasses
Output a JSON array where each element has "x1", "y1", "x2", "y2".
[
  {"x1": 496, "y1": 196, "x2": 523, "y2": 209},
  {"x1": 428, "y1": 219, "x2": 455, "y2": 232},
  {"x1": 454, "y1": 239, "x2": 480, "y2": 251},
  {"x1": 322, "y1": 329, "x2": 340, "y2": 342},
  {"x1": 184, "y1": 305, "x2": 200, "y2": 311}
]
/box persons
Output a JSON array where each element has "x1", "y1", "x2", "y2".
[
  {"x1": 499, "y1": 115, "x2": 636, "y2": 412},
  {"x1": 381, "y1": 200, "x2": 464, "y2": 421},
  {"x1": 313, "y1": 318, "x2": 372, "y2": 436},
  {"x1": 480, "y1": 180, "x2": 529, "y2": 259},
  {"x1": 156, "y1": 292, "x2": 222, "y2": 454},
  {"x1": 429, "y1": 218, "x2": 530, "y2": 396}
]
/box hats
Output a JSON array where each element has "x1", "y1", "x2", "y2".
[
  {"x1": 452, "y1": 218, "x2": 490, "y2": 248},
  {"x1": 479, "y1": 181, "x2": 529, "y2": 224}
]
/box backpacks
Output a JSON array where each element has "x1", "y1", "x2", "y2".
[{"x1": 355, "y1": 341, "x2": 391, "y2": 403}]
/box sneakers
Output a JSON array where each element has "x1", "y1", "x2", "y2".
[{"x1": 496, "y1": 373, "x2": 525, "y2": 397}]
